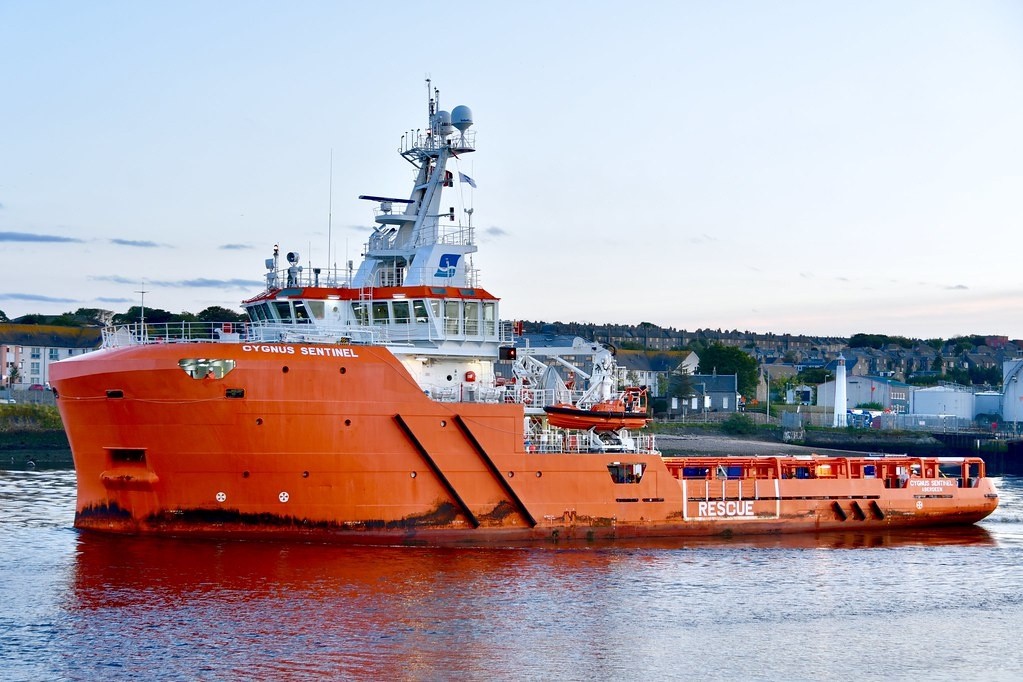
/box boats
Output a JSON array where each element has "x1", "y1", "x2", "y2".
[{"x1": 49, "y1": 78, "x2": 1001, "y2": 552}]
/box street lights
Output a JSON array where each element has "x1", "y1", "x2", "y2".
[
  {"x1": 824, "y1": 375, "x2": 829, "y2": 427},
  {"x1": 700, "y1": 383, "x2": 706, "y2": 424},
  {"x1": 938, "y1": 403, "x2": 945, "y2": 433},
  {"x1": 1013, "y1": 377, "x2": 1017, "y2": 432},
  {"x1": 132, "y1": 290, "x2": 150, "y2": 344},
  {"x1": 887, "y1": 379, "x2": 891, "y2": 414}
]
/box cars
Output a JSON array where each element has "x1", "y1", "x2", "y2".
[
  {"x1": 739, "y1": 402, "x2": 745, "y2": 406},
  {"x1": 45, "y1": 383, "x2": 51, "y2": 391},
  {"x1": 29, "y1": 385, "x2": 43, "y2": 391},
  {"x1": 9, "y1": 399, "x2": 16, "y2": 404}
]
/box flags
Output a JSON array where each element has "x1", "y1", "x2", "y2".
[
  {"x1": 443, "y1": 171, "x2": 454, "y2": 187},
  {"x1": 460, "y1": 173, "x2": 476, "y2": 188}
]
